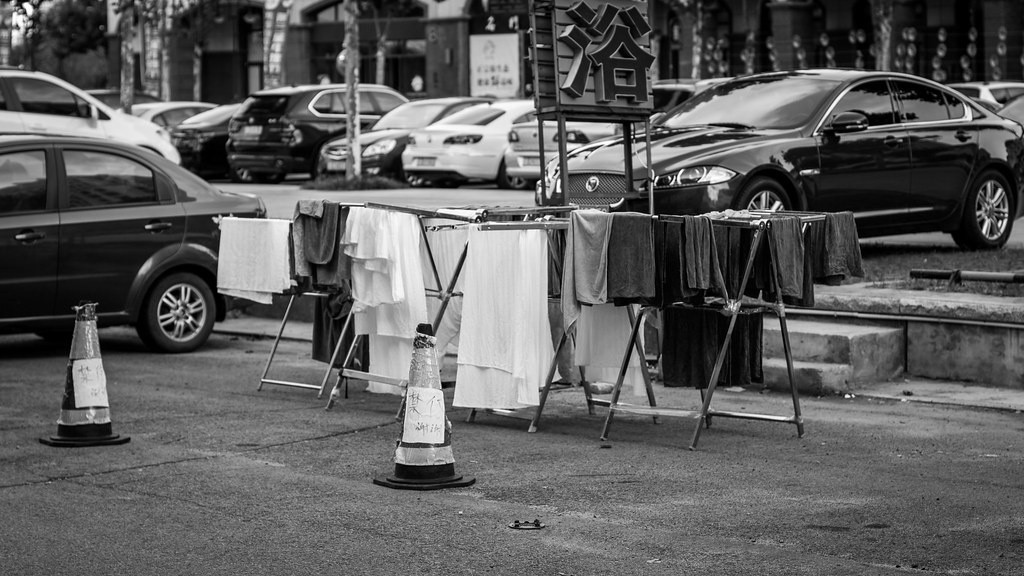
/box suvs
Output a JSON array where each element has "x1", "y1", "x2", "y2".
[
  {"x1": 226, "y1": 83, "x2": 410, "y2": 185},
  {"x1": 0, "y1": 66, "x2": 182, "y2": 167}
]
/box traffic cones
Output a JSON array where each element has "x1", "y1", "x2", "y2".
[
  {"x1": 39, "y1": 302, "x2": 130, "y2": 447},
  {"x1": 374, "y1": 323, "x2": 477, "y2": 491}
]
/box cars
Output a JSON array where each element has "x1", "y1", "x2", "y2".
[
  {"x1": 504, "y1": 78, "x2": 732, "y2": 177},
  {"x1": 318, "y1": 96, "x2": 498, "y2": 187},
  {"x1": 83, "y1": 89, "x2": 243, "y2": 179},
  {"x1": 0, "y1": 135, "x2": 267, "y2": 354},
  {"x1": 536, "y1": 69, "x2": 1024, "y2": 249},
  {"x1": 402, "y1": 100, "x2": 535, "y2": 189}
]
